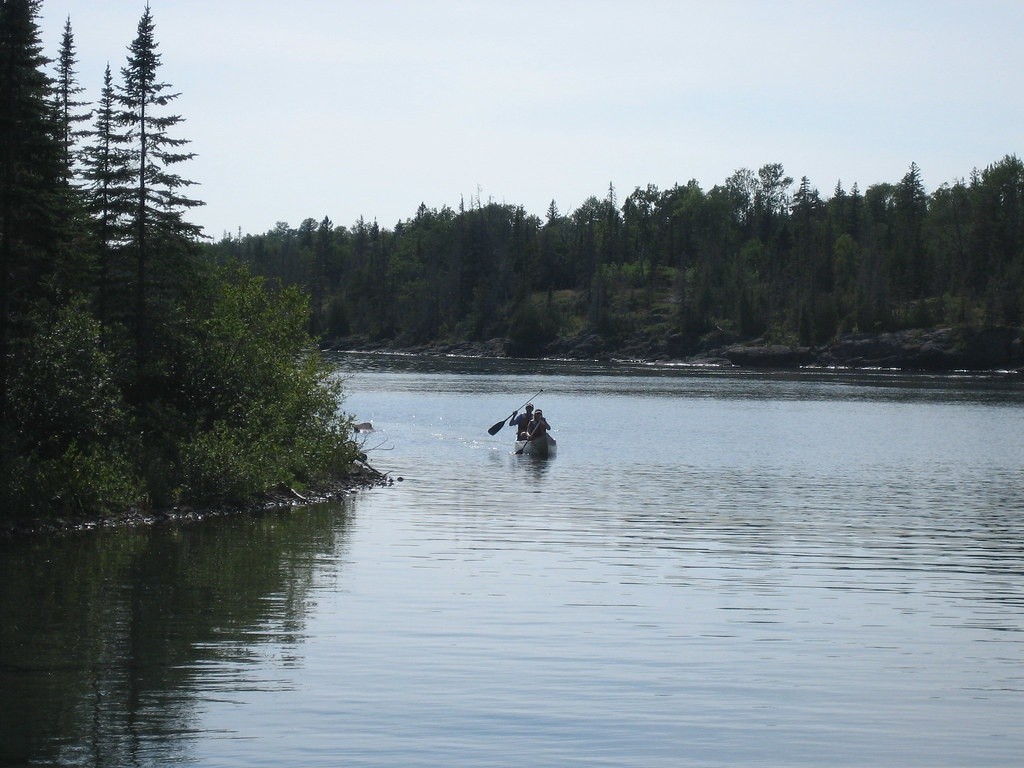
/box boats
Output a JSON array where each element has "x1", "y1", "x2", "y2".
[{"x1": 514, "y1": 433, "x2": 558, "y2": 458}]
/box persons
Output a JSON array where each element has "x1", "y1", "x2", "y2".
[{"x1": 509, "y1": 403, "x2": 551, "y2": 440}]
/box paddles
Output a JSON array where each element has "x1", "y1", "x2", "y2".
[
  {"x1": 515, "y1": 419, "x2": 543, "y2": 455},
  {"x1": 487, "y1": 389, "x2": 544, "y2": 436}
]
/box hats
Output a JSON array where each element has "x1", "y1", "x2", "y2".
[
  {"x1": 535, "y1": 411, "x2": 542, "y2": 413},
  {"x1": 526, "y1": 403, "x2": 533, "y2": 408}
]
